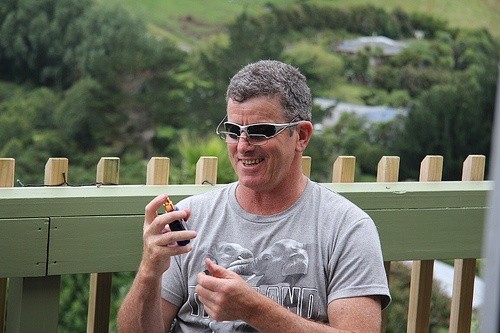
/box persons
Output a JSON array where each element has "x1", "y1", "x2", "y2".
[{"x1": 116, "y1": 59, "x2": 392, "y2": 333}]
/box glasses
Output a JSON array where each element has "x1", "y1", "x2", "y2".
[
  {"x1": 216, "y1": 114, "x2": 299, "y2": 146},
  {"x1": 223, "y1": 120, "x2": 306, "y2": 139}
]
